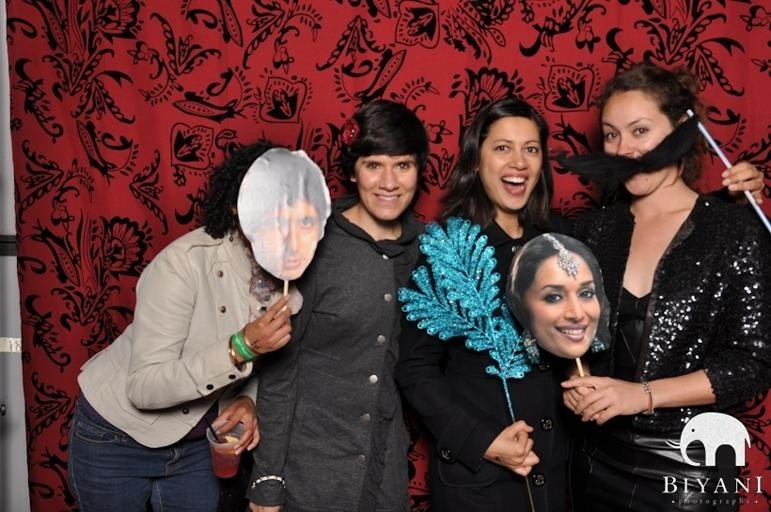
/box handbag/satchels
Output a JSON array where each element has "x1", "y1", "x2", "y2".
[{"x1": 216, "y1": 456, "x2": 251, "y2": 512}]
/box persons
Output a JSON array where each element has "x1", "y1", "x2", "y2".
[
  {"x1": 576, "y1": 62, "x2": 767, "y2": 508},
  {"x1": 397, "y1": 100, "x2": 587, "y2": 512},
  {"x1": 508, "y1": 232, "x2": 613, "y2": 376},
  {"x1": 69, "y1": 140, "x2": 332, "y2": 510},
  {"x1": 238, "y1": 145, "x2": 330, "y2": 287},
  {"x1": 249, "y1": 100, "x2": 424, "y2": 511}
]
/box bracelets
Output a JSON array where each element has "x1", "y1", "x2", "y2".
[
  {"x1": 229, "y1": 331, "x2": 259, "y2": 368},
  {"x1": 641, "y1": 380, "x2": 654, "y2": 415}
]
